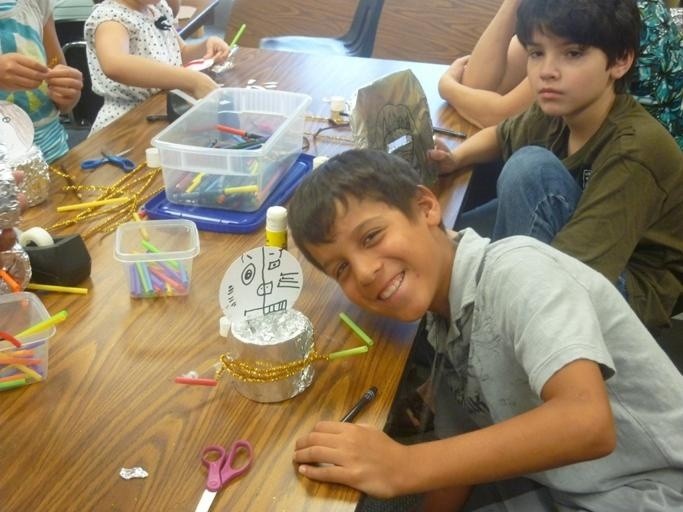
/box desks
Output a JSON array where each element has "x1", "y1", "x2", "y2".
[{"x1": 0, "y1": 45, "x2": 483, "y2": 512}]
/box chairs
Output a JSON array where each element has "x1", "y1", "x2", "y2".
[
  {"x1": 53, "y1": 16, "x2": 105, "y2": 129},
  {"x1": 257, "y1": 0, "x2": 384, "y2": 59}
]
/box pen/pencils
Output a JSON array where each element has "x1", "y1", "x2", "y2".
[
  {"x1": 341, "y1": 387, "x2": 377, "y2": 423},
  {"x1": 432, "y1": 127, "x2": 466, "y2": 139},
  {"x1": 227, "y1": 24, "x2": 246, "y2": 50},
  {"x1": 172, "y1": 124, "x2": 297, "y2": 210}
]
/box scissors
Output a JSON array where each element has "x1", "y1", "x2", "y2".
[
  {"x1": 81, "y1": 148, "x2": 135, "y2": 172},
  {"x1": 195, "y1": 440, "x2": 253, "y2": 512}
]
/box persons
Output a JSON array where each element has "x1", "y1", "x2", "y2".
[
  {"x1": 286, "y1": 147, "x2": 683, "y2": 512},
  {"x1": 84, "y1": 0, "x2": 230, "y2": 140},
  {"x1": 396, "y1": 0, "x2": 683, "y2": 432},
  {"x1": 0, "y1": 0, "x2": 84, "y2": 164},
  {"x1": 439, "y1": 0, "x2": 683, "y2": 151}
]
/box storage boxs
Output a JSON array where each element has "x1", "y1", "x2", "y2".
[{"x1": 140, "y1": 85, "x2": 314, "y2": 233}]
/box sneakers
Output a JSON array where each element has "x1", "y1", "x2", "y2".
[{"x1": 387, "y1": 391, "x2": 435, "y2": 435}]
